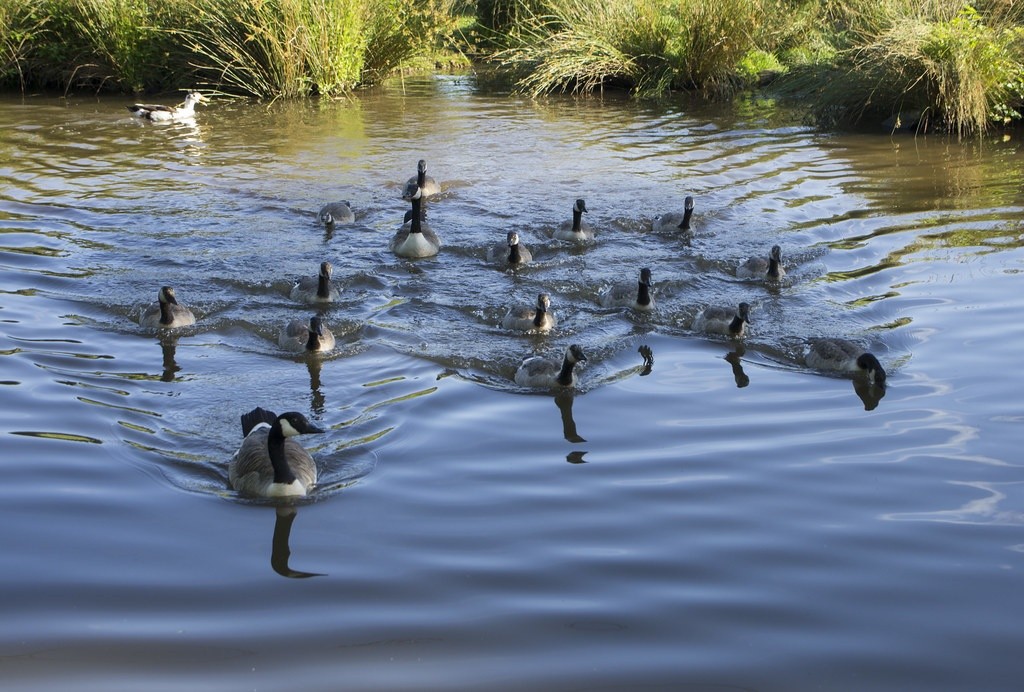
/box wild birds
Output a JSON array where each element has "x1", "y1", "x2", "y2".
[
  {"x1": 124, "y1": 92, "x2": 211, "y2": 127},
  {"x1": 227, "y1": 407, "x2": 326, "y2": 498},
  {"x1": 803, "y1": 335, "x2": 887, "y2": 391},
  {"x1": 137, "y1": 286, "x2": 197, "y2": 329},
  {"x1": 277, "y1": 160, "x2": 440, "y2": 356},
  {"x1": 435, "y1": 59, "x2": 478, "y2": 87},
  {"x1": 485, "y1": 196, "x2": 790, "y2": 391}
]
